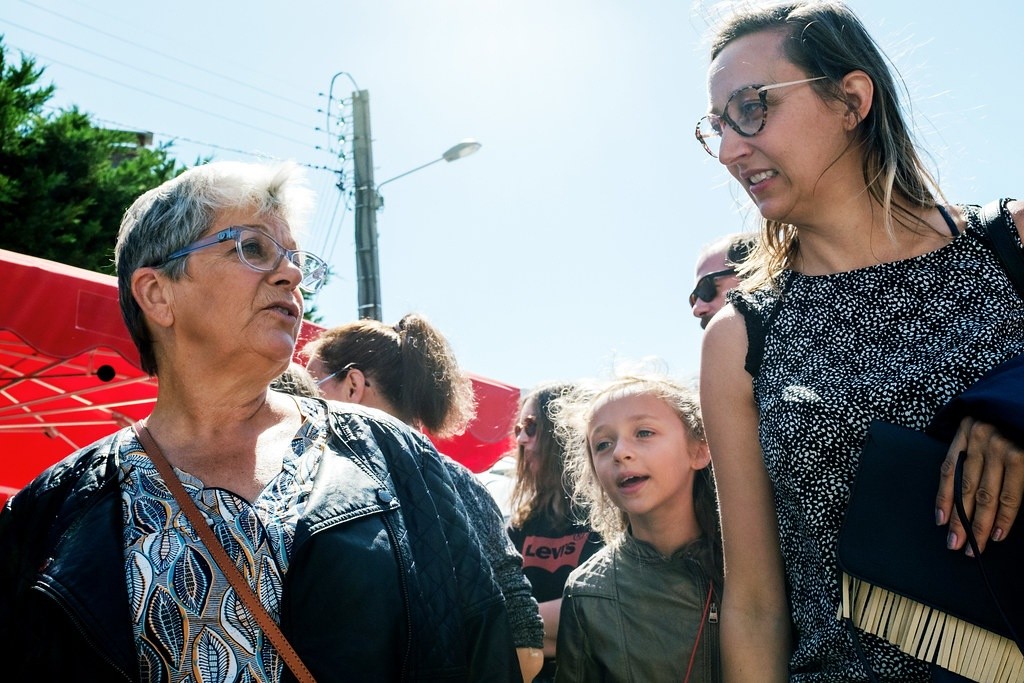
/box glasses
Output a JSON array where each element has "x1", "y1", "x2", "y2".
[
  {"x1": 147, "y1": 226, "x2": 330, "y2": 293},
  {"x1": 687, "y1": 264, "x2": 739, "y2": 308},
  {"x1": 515, "y1": 415, "x2": 536, "y2": 439},
  {"x1": 695, "y1": 76, "x2": 828, "y2": 159}
]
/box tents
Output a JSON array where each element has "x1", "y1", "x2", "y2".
[{"x1": 1, "y1": 244, "x2": 522, "y2": 513}]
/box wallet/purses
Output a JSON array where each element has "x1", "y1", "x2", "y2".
[{"x1": 836, "y1": 416, "x2": 1024, "y2": 646}]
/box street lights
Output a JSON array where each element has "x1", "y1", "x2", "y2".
[{"x1": 356, "y1": 141, "x2": 483, "y2": 326}]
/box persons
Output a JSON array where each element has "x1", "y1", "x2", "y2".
[
  {"x1": 700, "y1": 1, "x2": 1024, "y2": 683},
  {"x1": 555, "y1": 377, "x2": 725, "y2": 683},
  {"x1": 0, "y1": 162, "x2": 524, "y2": 683},
  {"x1": 507, "y1": 387, "x2": 608, "y2": 683},
  {"x1": 306, "y1": 313, "x2": 547, "y2": 683},
  {"x1": 689, "y1": 232, "x2": 769, "y2": 329}
]
